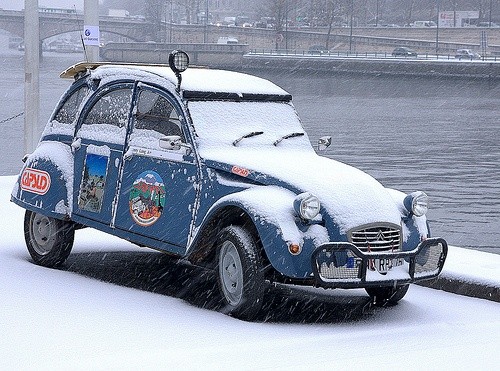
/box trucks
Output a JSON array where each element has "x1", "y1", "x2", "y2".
[
  {"x1": 108, "y1": 9, "x2": 129, "y2": 17},
  {"x1": 217, "y1": 37, "x2": 239, "y2": 45}
]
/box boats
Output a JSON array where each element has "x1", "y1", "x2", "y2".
[{"x1": 8, "y1": 32, "x2": 115, "y2": 53}]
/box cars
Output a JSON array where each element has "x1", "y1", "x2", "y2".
[
  {"x1": 253, "y1": 17, "x2": 359, "y2": 29},
  {"x1": 455, "y1": 49, "x2": 481, "y2": 60},
  {"x1": 308, "y1": 45, "x2": 330, "y2": 55},
  {"x1": 367, "y1": 24, "x2": 401, "y2": 28},
  {"x1": 392, "y1": 47, "x2": 417, "y2": 57},
  {"x1": 404, "y1": 24, "x2": 414, "y2": 28},
  {"x1": 477, "y1": 21, "x2": 499, "y2": 27},
  {"x1": 9, "y1": 49, "x2": 448, "y2": 321},
  {"x1": 414, "y1": 21, "x2": 438, "y2": 28},
  {"x1": 169, "y1": 10, "x2": 253, "y2": 27}
]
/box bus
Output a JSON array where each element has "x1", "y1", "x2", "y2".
[{"x1": 38, "y1": 8, "x2": 75, "y2": 14}]
